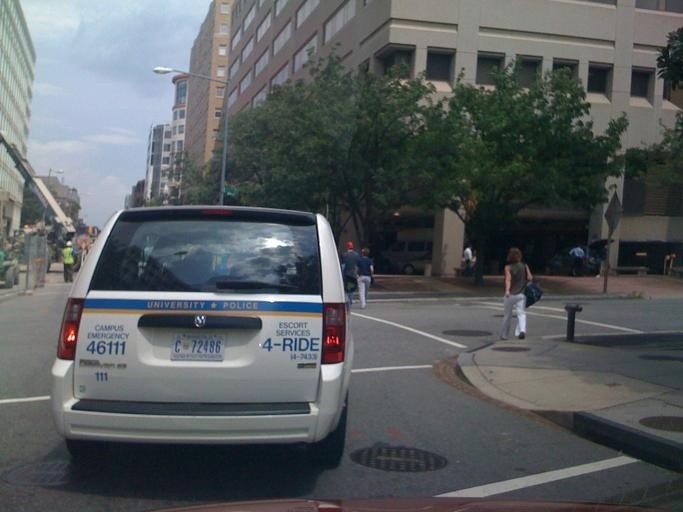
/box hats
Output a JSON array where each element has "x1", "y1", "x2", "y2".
[{"x1": 347, "y1": 243, "x2": 353, "y2": 249}]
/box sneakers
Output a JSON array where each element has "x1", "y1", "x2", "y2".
[{"x1": 519, "y1": 332, "x2": 524, "y2": 339}]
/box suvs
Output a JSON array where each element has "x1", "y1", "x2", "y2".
[{"x1": 49, "y1": 205, "x2": 355, "y2": 469}]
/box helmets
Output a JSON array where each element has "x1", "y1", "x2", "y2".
[{"x1": 66, "y1": 241, "x2": 72, "y2": 246}]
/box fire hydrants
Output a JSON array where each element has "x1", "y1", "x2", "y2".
[{"x1": 563, "y1": 303, "x2": 583, "y2": 341}]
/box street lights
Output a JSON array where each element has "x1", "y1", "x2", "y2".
[
  {"x1": 151, "y1": 65, "x2": 230, "y2": 206},
  {"x1": 40, "y1": 167, "x2": 65, "y2": 222}
]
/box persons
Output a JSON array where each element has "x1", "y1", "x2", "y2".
[
  {"x1": 60, "y1": 241, "x2": 77, "y2": 283},
  {"x1": 462, "y1": 245, "x2": 474, "y2": 276},
  {"x1": 353, "y1": 246, "x2": 373, "y2": 310},
  {"x1": 499, "y1": 248, "x2": 533, "y2": 340},
  {"x1": 566, "y1": 242, "x2": 608, "y2": 278},
  {"x1": 338, "y1": 242, "x2": 359, "y2": 309}
]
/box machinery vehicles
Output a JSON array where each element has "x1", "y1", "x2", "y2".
[
  {"x1": 0, "y1": 129, "x2": 98, "y2": 272},
  {"x1": 0, "y1": 183, "x2": 20, "y2": 289}
]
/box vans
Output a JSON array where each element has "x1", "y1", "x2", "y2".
[{"x1": 370, "y1": 227, "x2": 431, "y2": 275}]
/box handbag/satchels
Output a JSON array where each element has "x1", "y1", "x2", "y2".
[{"x1": 525, "y1": 284, "x2": 542, "y2": 307}]
